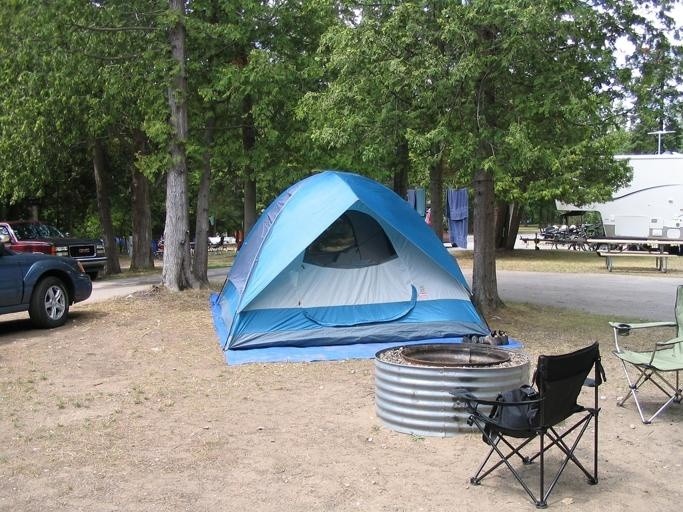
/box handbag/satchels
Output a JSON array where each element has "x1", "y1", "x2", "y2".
[{"x1": 491, "y1": 384, "x2": 539, "y2": 430}]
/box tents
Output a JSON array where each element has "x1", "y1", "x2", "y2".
[{"x1": 212, "y1": 171, "x2": 521, "y2": 365}]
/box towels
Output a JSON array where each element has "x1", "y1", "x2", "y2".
[
  {"x1": 446, "y1": 188, "x2": 470, "y2": 249},
  {"x1": 407, "y1": 188, "x2": 426, "y2": 216}
]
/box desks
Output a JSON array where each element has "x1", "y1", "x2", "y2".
[{"x1": 586, "y1": 239, "x2": 683, "y2": 252}]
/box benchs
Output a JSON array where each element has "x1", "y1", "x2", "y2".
[
  {"x1": 523, "y1": 234, "x2": 598, "y2": 253},
  {"x1": 596, "y1": 249, "x2": 677, "y2": 273}
]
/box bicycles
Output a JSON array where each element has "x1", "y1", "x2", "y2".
[{"x1": 538, "y1": 218, "x2": 611, "y2": 252}]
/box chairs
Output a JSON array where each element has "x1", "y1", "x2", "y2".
[
  {"x1": 608, "y1": 285, "x2": 683, "y2": 424},
  {"x1": 450, "y1": 341, "x2": 606, "y2": 509}
]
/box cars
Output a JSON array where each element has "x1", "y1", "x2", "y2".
[
  {"x1": 0, "y1": 239, "x2": 94, "y2": 329},
  {"x1": 0, "y1": 220, "x2": 108, "y2": 279}
]
